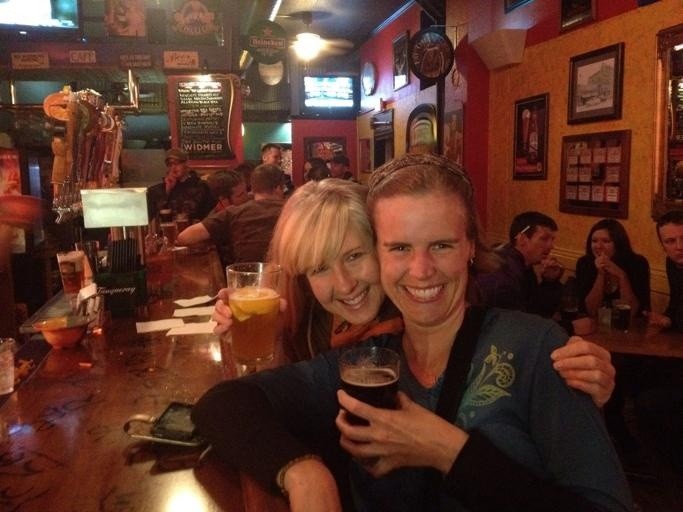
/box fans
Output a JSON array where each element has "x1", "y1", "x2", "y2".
[{"x1": 281, "y1": 9, "x2": 354, "y2": 55}]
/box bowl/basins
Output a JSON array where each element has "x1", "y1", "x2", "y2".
[
  {"x1": 31, "y1": 313, "x2": 97, "y2": 351},
  {"x1": 1, "y1": 193, "x2": 46, "y2": 229}
]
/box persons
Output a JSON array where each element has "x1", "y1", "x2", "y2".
[
  {"x1": 469, "y1": 210, "x2": 596, "y2": 336},
  {"x1": 574, "y1": 219, "x2": 651, "y2": 317},
  {"x1": 189, "y1": 154, "x2": 636, "y2": 511},
  {"x1": 211, "y1": 176, "x2": 617, "y2": 410},
  {"x1": 146, "y1": 143, "x2": 362, "y2": 287},
  {"x1": 642, "y1": 210, "x2": 683, "y2": 332}
]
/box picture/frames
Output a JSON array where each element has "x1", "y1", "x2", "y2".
[
  {"x1": 388, "y1": 29, "x2": 411, "y2": 93},
  {"x1": 299, "y1": 135, "x2": 347, "y2": 164},
  {"x1": 564, "y1": 39, "x2": 627, "y2": 127},
  {"x1": 359, "y1": 59, "x2": 377, "y2": 100},
  {"x1": 510, "y1": 91, "x2": 550, "y2": 182},
  {"x1": 498, "y1": 0, "x2": 665, "y2": 34},
  {"x1": 557, "y1": 127, "x2": 632, "y2": 220},
  {"x1": 647, "y1": 17, "x2": 682, "y2": 225}
]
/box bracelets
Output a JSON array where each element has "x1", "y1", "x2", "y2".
[
  {"x1": 619, "y1": 271, "x2": 626, "y2": 282},
  {"x1": 279, "y1": 454, "x2": 325, "y2": 497}
]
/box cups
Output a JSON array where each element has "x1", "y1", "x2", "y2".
[
  {"x1": 75, "y1": 240, "x2": 99, "y2": 276},
  {"x1": 333, "y1": 342, "x2": 404, "y2": 474},
  {"x1": 614, "y1": 304, "x2": 631, "y2": 334},
  {"x1": 563, "y1": 308, "x2": 579, "y2": 333},
  {"x1": 158, "y1": 209, "x2": 189, "y2": 250},
  {"x1": 598, "y1": 308, "x2": 612, "y2": 339},
  {"x1": 223, "y1": 261, "x2": 283, "y2": 365},
  {"x1": 56, "y1": 250, "x2": 86, "y2": 312},
  {"x1": 0, "y1": 335, "x2": 16, "y2": 397}
]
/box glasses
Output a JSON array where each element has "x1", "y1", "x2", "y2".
[{"x1": 166, "y1": 161, "x2": 183, "y2": 166}]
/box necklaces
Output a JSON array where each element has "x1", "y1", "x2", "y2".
[{"x1": 608, "y1": 279, "x2": 617, "y2": 285}]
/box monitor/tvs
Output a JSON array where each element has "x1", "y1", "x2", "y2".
[
  {"x1": 301, "y1": 75, "x2": 360, "y2": 121},
  {"x1": 0, "y1": 0, "x2": 84, "y2": 43}
]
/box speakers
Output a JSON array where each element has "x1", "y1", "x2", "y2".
[{"x1": 145, "y1": 9, "x2": 168, "y2": 43}]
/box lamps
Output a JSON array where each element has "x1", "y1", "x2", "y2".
[{"x1": 289, "y1": 33, "x2": 323, "y2": 63}]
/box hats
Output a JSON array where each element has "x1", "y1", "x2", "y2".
[
  {"x1": 165, "y1": 147, "x2": 186, "y2": 160},
  {"x1": 327, "y1": 155, "x2": 349, "y2": 167}
]
